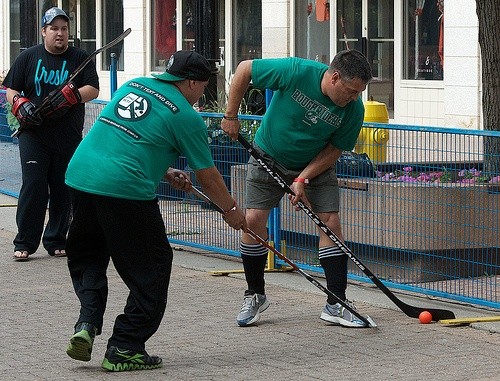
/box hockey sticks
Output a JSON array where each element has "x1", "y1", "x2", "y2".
[
  {"x1": 236, "y1": 131, "x2": 457, "y2": 322},
  {"x1": 10, "y1": 28, "x2": 132, "y2": 138},
  {"x1": 190, "y1": 185, "x2": 379, "y2": 328}
]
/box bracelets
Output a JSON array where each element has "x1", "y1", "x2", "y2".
[
  {"x1": 225, "y1": 200, "x2": 237, "y2": 213},
  {"x1": 294, "y1": 178, "x2": 309, "y2": 184},
  {"x1": 223, "y1": 115, "x2": 238, "y2": 120}
]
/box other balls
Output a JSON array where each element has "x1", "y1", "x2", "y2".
[{"x1": 419, "y1": 311, "x2": 432, "y2": 324}]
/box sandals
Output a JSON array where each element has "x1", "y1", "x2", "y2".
[
  {"x1": 13, "y1": 248, "x2": 29, "y2": 259},
  {"x1": 51, "y1": 248, "x2": 67, "y2": 256}
]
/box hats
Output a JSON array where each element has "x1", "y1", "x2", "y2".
[
  {"x1": 151, "y1": 50, "x2": 210, "y2": 82},
  {"x1": 41, "y1": 7, "x2": 68, "y2": 26}
]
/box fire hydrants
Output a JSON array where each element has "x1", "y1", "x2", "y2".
[{"x1": 353, "y1": 95, "x2": 390, "y2": 163}]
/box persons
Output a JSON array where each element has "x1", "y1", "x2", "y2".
[
  {"x1": 220, "y1": 49, "x2": 373, "y2": 327},
  {"x1": 65, "y1": 52, "x2": 248, "y2": 371},
  {"x1": 2, "y1": 7, "x2": 99, "y2": 258}
]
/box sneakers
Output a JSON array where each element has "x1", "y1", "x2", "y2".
[
  {"x1": 65, "y1": 322, "x2": 95, "y2": 361},
  {"x1": 320, "y1": 299, "x2": 367, "y2": 327},
  {"x1": 101, "y1": 337, "x2": 162, "y2": 372},
  {"x1": 236, "y1": 290, "x2": 270, "y2": 325}
]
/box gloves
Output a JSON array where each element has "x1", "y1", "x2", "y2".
[
  {"x1": 11, "y1": 94, "x2": 42, "y2": 126},
  {"x1": 41, "y1": 82, "x2": 82, "y2": 122}
]
